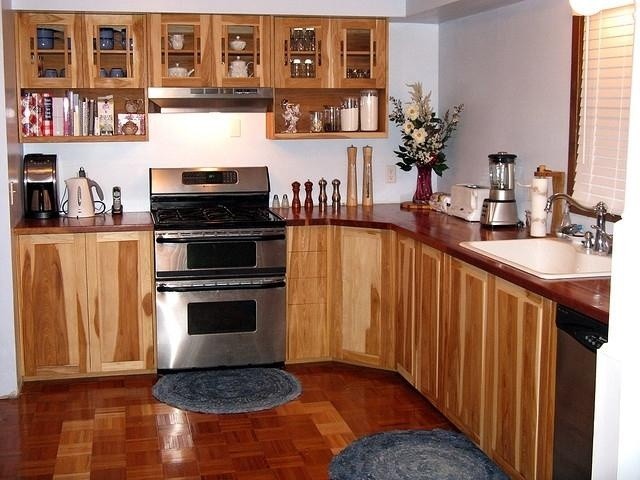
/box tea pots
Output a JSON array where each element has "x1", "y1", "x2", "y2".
[
  {"x1": 168, "y1": 63, "x2": 194, "y2": 77},
  {"x1": 124, "y1": 98, "x2": 143, "y2": 113},
  {"x1": 228, "y1": 57, "x2": 253, "y2": 77}
]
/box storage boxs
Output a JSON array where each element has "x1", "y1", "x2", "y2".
[{"x1": 117, "y1": 113, "x2": 146, "y2": 136}]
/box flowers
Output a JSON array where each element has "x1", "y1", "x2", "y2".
[{"x1": 389, "y1": 82, "x2": 465, "y2": 179}]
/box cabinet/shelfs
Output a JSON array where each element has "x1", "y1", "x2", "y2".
[
  {"x1": 397, "y1": 234, "x2": 446, "y2": 414},
  {"x1": 264, "y1": 17, "x2": 390, "y2": 141},
  {"x1": 285, "y1": 224, "x2": 395, "y2": 370},
  {"x1": 139, "y1": 12, "x2": 271, "y2": 87},
  {"x1": 14, "y1": 10, "x2": 151, "y2": 143},
  {"x1": 445, "y1": 256, "x2": 555, "y2": 480},
  {"x1": 15, "y1": 227, "x2": 155, "y2": 383}
]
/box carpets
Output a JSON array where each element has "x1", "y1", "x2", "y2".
[
  {"x1": 151, "y1": 365, "x2": 305, "y2": 414},
  {"x1": 326, "y1": 428, "x2": 512, "y2": 480}
]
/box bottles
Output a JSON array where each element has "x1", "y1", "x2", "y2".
[
  {"x1": 319, "y1": 178, "x2": 327, "y2": 203},
  {"x1": 272, "y1": 194, "x2": 279, "y2": 208},
  {"x1": 281, "y1": 194, "x2": 289, "y2": 209},
  {"x1": 310, "y1": 111, "x2": 324, "y2": 133},
  {"x1": 292, "y1": 181, "x2": 302, "y2": 209},
  {"x1": 303, "y1": 179, "x2": 313, "y2": 207},
  {"x1": 291, "y1": 28, "x2": 315, "y2": 51},
  {"x1": 290, "y1": 58, "x2": 315, "y2": 77},
  {"x1": 332, "y1": 178, "x2": 341, "y2": 202},
  {"x1": 324, "y1": 104, "x2": 341, "y2": 131}
]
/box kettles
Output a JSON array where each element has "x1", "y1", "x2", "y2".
[{"x1": 63, "y1": 177, "x2": 104, "y2": 217}]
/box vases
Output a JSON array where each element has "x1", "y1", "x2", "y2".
[{"x1": 410, "y1": 159, "x2": 436, "y2": 205}]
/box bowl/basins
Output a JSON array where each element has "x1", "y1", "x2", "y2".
[
  {"x1": 37, "y1": 29, "x2": 54, "y2": 37},
  {"x1": 38, "y1": 37, "x2": 54, "y2": 49},
  {"x1": 94, "y1": 28, "x2": 114, "y2": 39},
  {"x1": 94, "y1": 39, "x2": 114, "y2": 50}
]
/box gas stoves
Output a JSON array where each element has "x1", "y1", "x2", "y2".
[{"x1": 148, "y1": 194, "x2": 287, "y2": 227}]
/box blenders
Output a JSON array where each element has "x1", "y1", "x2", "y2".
[{"x1": 480, "y1": 152, "x2": 520, "y2": 229}]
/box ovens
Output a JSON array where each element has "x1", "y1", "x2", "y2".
[{"x1": 155, "y1": 229, "x2": 288, "y2": 373}]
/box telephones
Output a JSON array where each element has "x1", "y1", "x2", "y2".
[{"x1": 111, "y1": 185, "x2": 124, "y2": 216}]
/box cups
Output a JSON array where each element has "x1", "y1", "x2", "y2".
[
  {"x1": 110, "y1": 67, "x2": 124, "y2": 77},
  {"x1": 101, "y1": 68, "x2": 106, "y2": 77},
  {"x1": 59, "y1": 68, "x2": 65, "y2": 77},
  {"x1": 167, "y1": 35, "x2": 185, "y2": 50},
  {"x1": 43, "y1": 68, "x2": 58, "y2": 77}
]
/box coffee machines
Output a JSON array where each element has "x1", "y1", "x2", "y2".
[{"x1": 23, "y1": 153, "x2": 60, "y2": 220}]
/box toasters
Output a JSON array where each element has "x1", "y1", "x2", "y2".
[{"x1": 448, "y1": 182, "x2": 490, "y2": 223}]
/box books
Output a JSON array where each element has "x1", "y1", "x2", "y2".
[{"x1": 21, "y1": 91, "x2": 99, "y2": 137}]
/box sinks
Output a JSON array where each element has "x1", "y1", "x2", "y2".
[{"x1": 459, "y1": 238, "x2": 612, "y2": 279}]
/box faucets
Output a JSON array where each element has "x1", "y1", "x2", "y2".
[{"x1": 541, "y1": 192, "x2": 608, "y2": 253}]
